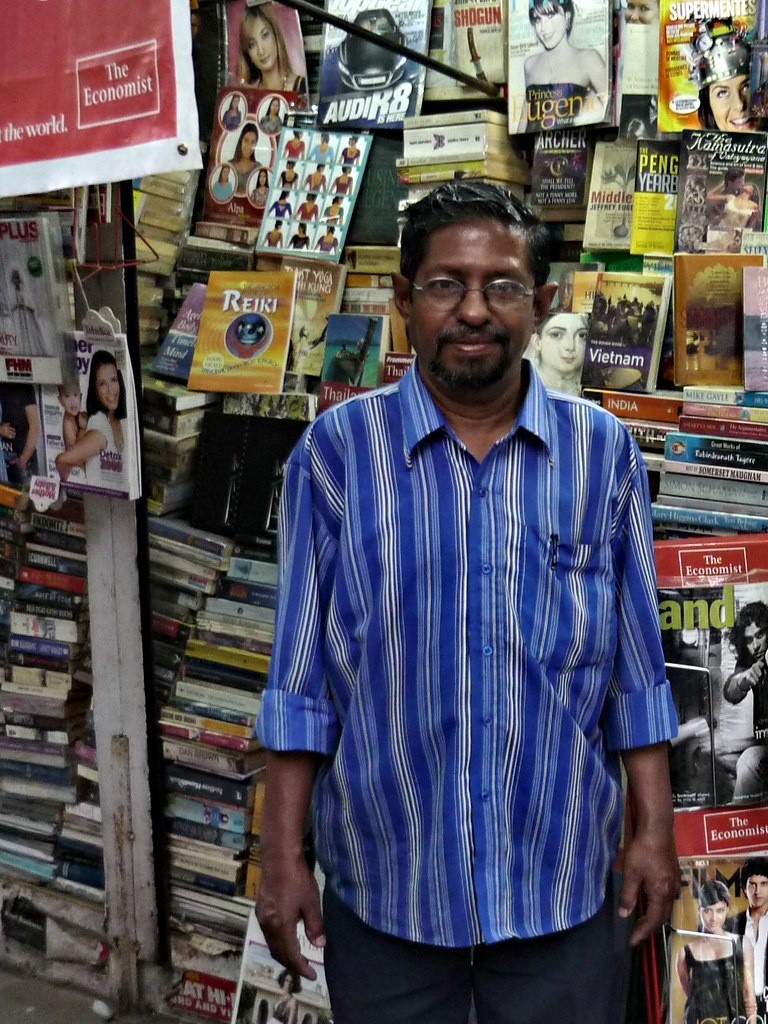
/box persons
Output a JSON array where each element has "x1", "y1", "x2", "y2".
[
  {"x1": 0, "y1": 382, "x2": 41, "y2": 488},
  {"x1": 57, "y1": 381, "x2": 87, "y2": 477},
  {"x1": 624, "y1": 0, "x2": 659, "y2": 25},
  {"x1": 517, "y1": 0, "x2": 607, "y2": 135},
  {"x1": 676, "y1": 878, "x2": 758, "y2": 1024},
  {"x1": 236, "y1": 5, "x2": 307, "y2": 96},
  {"x1": 55, "y1": 349, "x2": 130, "y2": 493},
  {"x1": 228, "y1": 124, "x2": 262, "y2": 196},
  {"x1": 707, "y1": 181, "x2": 759, "y2": 230},
  {"x1": 262, "y1": 130, "x2": 360, "y2": 256},
  {"x1": 273, "y1": 969, "x2": 303, "y2": 1024},
  {"x1": 223, "y1": 94, "x2": 242, "y2": 130},
  {"x1": 259, "y1": 97, "x2": 283, "y2": 135},
  {"x1": 251, "y1": 177, "x2": 681, "y2": 1024},
  {"x1": 698, "y1": 36, "x2": 763, "y2": 131},
  {"x1": 704, "y1": 167, "x2": 759, "y2": 230},
  {"x1": 721, "y1": 600, "x2": 767, "y2": 807},
  {"x1": 725, "y1": 856, "x2": 768, "y2": 1024},
  {"x1": 251, "y1": 169, "x2": 269, "y2": 207},
  {"x1": 212, "y1": 166, "x2": 234, "y2": 202}
]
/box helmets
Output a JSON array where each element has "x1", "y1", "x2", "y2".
[{"x1": 702, "y1": 39, "x2": 751, "y2": 88}]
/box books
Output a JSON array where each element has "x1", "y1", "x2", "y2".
[
  {"x1": 0, "y1": 171, "x2": 767, "y2": 1024},
  {"x1": 315, "y1": 1, "x2": 768, "y2": 256},
  {"x1": 221, "y1": 0, "x2": 310, "y2": 115},
  {"x1": 255, "y1": 128, "x2": 374, "y2": 266}
]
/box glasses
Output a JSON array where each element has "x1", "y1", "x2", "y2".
[{"x1": 412, "y1": 277, "x2": 534, "y2": 310}]
